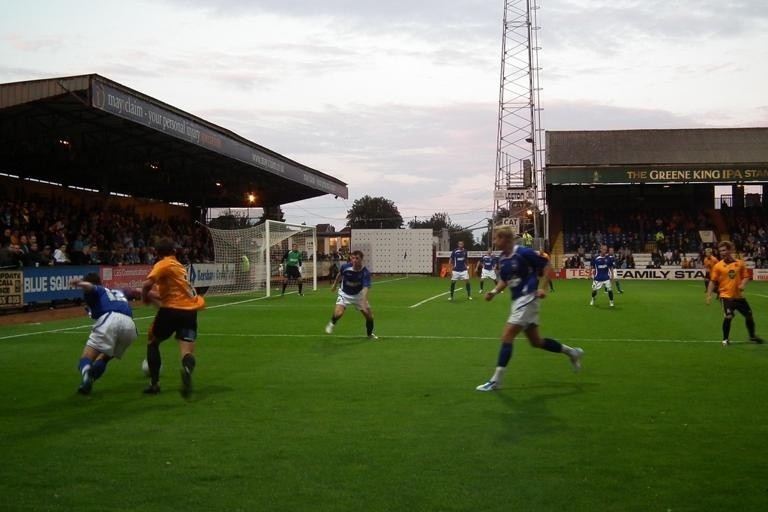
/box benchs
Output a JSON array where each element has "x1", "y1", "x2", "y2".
[{"x1": 563, "y1": 251, "x2": 700, "y2": 269}]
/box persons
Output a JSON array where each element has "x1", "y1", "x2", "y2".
[
  {"x1": 590, "y1": 244, "x2": 614, "y2": 307},
  {"x1": 71, "y1": 272, "x2": 159, "y2": 398},
  {"x1": 604, "y1": 247, "x2": 624, "y2": 294},
  {"x1": 140, "y1": 237, "x2": 205, "y2": 397},
  {"x1": 566, "y1": 201, "x2": 768, "y2": 268},
  {"x1": 325, "y1": 250, "x2": 378, "y2": 339},
  {"x1": 475, "y1": 248, "x2": 504, "y2": 294},
  {"x1": 448, "y1": 241, "x2": 473, "y2": 301},
  {"x1": 706, "y1": 241, "x2": 764, "y2": 346},
  {"x1": 539, "y1": 248, "x2": 554, "y2": 292},
  {"x1": 523, "y1": 230, "x2": 533, "y2": 246},
  {"x1": 476, "y1": 225, "x2": 585, "y2": 391},
  {"x1": 238, "y1": 250, "x2": 249, "y2": 291},
  {"x1": 1, "y1": 193, "x2": 351, "y2": 268},
  {"x1": 329, "y1": 264, "x2": 339, "y2": 285},
  {"x1": 703, "y1": 248, "x2": 720, "y2": 299},
  {"x1": 279, "y1": 244, "x2": 304, "y2": 297}
]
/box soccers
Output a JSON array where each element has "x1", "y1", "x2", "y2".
[{"x1": 142, "y1": 360, "x2": 151, "y2": 375}]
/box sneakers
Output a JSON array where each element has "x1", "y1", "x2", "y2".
[
  {"x1": 179, "y1": 365, "x2": 193, "y2": 399},
  {"x1": 367, "y1": 333, "x2": 378, "y2": 341},
  {"x1": 143, "y1": 378, "x2": 160, "y2": 394},
  {"x1": 609, "y1": 300, "x2": 614, "y2": 307},
  {"x1": 590, "y1": 299, "x2": 595, "y2": 306},
  {"x1": 721, "y1": 339, "x2": 729, "y2": 347},
  {"x1": 78, "y1": 369, "x2": 95, "y2": 395},
  {"x1": 476, "y1": 378, "x2": 503, "y2": 392},
  {"x1": 749, "y1": 338, "x2": 765, "y2": 344},
  {"x1": 570, "y1": 347, "x2": 584, "y2": 373},
  {"x1": 325, "y1": 321, "x2": 335, "y2": 334},
  {"x1": 617, "y1": 289, "x2": 624, "y2": 294}
]
